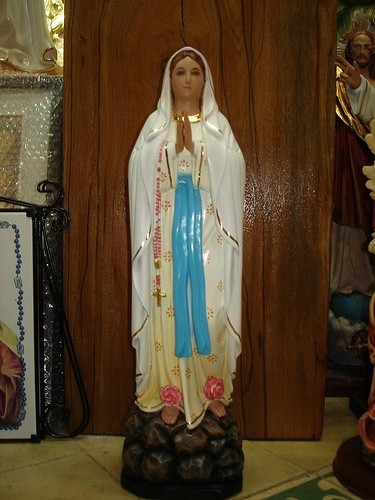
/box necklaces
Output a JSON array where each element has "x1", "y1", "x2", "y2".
[{"x1": 169, "y1": 111, "x2": 203, "y2": 124}]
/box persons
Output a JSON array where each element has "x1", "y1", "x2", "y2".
[
  {"x1": 126, "y1": 46, "x2": 247, "y2": 428},
  {"x1": 335, "y1": 54, "x2": 373, "y2": 135},
  {"x1": 326, "y1": 22, "x2": 373, "y2": 294}
]
